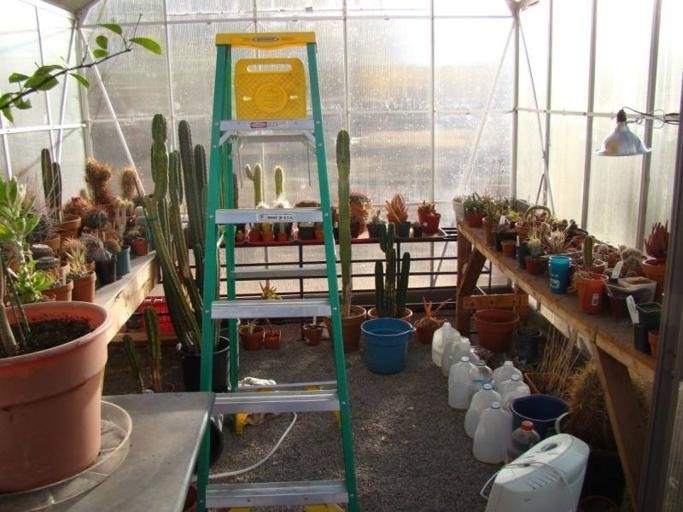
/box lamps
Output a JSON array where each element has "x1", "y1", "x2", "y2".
[{"x1": 603, "y1": 110, "x2": 682, "y2": 157}]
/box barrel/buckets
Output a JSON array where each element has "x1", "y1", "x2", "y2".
[
  {"x1": 361, "y1": 318, "x2": 415, "y2": 374},
  {"x1": 432, "y1": 322, "x2": 569, "y2": 464}
]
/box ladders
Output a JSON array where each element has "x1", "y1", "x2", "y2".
[{"x1": 197, "y1": 31, "x2": 358, "y2": 512}]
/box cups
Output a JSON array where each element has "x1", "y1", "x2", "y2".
[{"x1": 547, "y1": 256, "x2": 571, "y2": 294}]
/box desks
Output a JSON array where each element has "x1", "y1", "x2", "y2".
[
  {"x1": 0, "y1": 391, "x2": 216, "y2": 512},
  {"x1": 455, "y1": 220, "x2": 683, "y2": 512}
]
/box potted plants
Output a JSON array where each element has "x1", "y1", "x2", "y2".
[
  {"x1": 0, "y1": 114, "x2": 232, "y2": 493},
  {"x1": 220, "y1": 160, "x2": 442, "y2": 243},
  {"x1": 236, "y1": 283, "x2": 454, "y2": 373},
  {"x1": 369, "y1": 223, "x2": 413, "y2": 322},
  {"x1": 452, "y1": 193, "x2": 675, "y2": 491}
]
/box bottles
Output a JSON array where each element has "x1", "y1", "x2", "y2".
[{"x1": 506, "y1": 421, "x2": 541, "y2": 463}]
